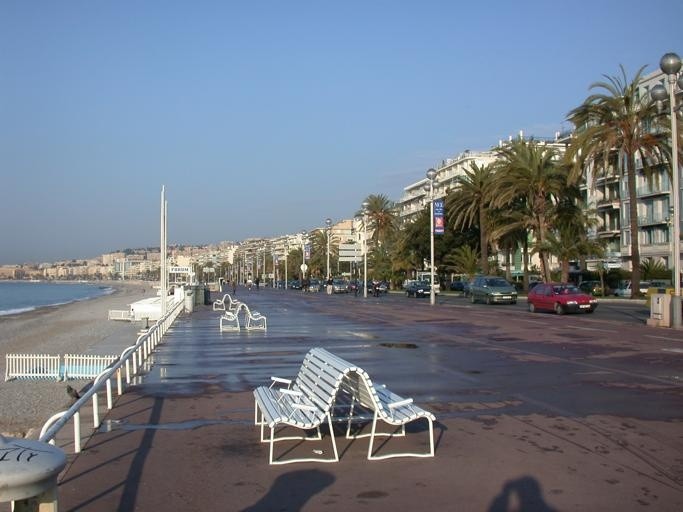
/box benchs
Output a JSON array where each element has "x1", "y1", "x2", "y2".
[
  {"x1": 212, "y1": 292, "x2": 268, "y2": 336},
  {"x1": 252, "y1": 346, "x2": 437, "y2": 466}
]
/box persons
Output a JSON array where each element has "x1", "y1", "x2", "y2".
[
  {"x1": 372, "y1": 280, "x2": 381, "y2": 296},
  {"x1": 326, "y1": 273, "x2": 333, "y2": 296},
  {"x1": 231, "y1": 278, "x2": 236, "y2": 295},
  {"x1": 254, "y1": 278, "x2": 259, "y2": 289}
]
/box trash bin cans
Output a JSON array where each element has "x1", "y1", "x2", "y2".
[{"x1": 184, "y1": 290, "x2": 194, "y2": 313}]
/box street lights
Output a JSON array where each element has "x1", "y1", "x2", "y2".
[
  {"x1": 361, "y1": 199, "x2": 371, "y2": 299},
  {"x1": 301, "y1": 230, "x2": 307, "y2": 278},
  {"x1": 424, "y1": 169, "x2": 439, "y2": 307},
  {"x1": 650, "y1": 52, "x2": 683, "y2": 330},
  {"x1": 324, "y1": 218, "x2": 333, "y2": 280}
]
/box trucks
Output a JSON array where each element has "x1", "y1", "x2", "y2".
[{"x1": 403, "y1": 271, "x2": 440, "y2": 295}]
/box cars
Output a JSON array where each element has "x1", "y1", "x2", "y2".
[
  {"x1": 578, "y1": 280, "x2": 612, "y2": 297},
  {"x1": 272, "y1": 278, "x2": 388, "y2": 297},
  {"x1": 405, "y1": 280, "x2": 437, "y2": 297},
  {"x1": 614, "y1": 281, "x2": 674, "y2": 299},
  {"x1": 449, "y1": 280, "x2": 466, "y2": 291},
  {"x1": 468, "y1": 274, "x2": 519, "y2": 304},
  {"x1": 527, "y1": 280, "x2": 599, "y2": 315}
]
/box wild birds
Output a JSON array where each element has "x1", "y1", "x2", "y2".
[{"x1": 66, "y1": 385, "x2": 80, "y2": 399}]
